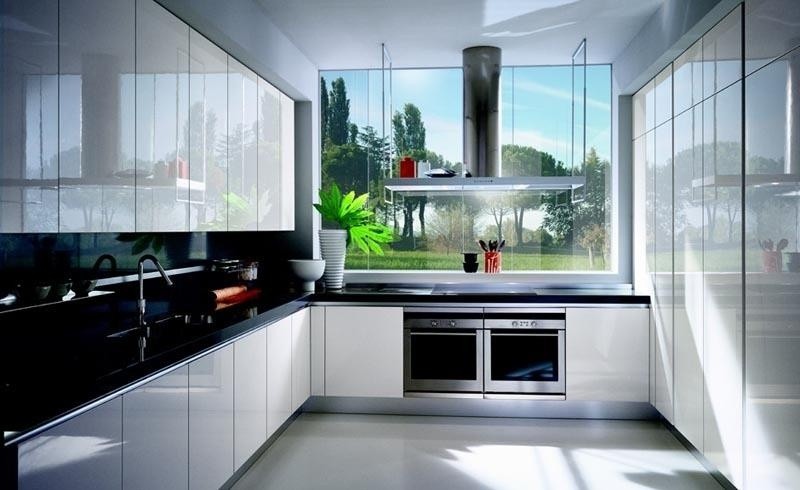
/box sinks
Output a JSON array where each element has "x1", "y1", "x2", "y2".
[{"x1": 106, "y1": 312, "x2": 215, "y2": 338}]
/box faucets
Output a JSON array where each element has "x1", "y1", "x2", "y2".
[
  {"x1": 137, "y1": 253, "x2": 175, "y2": 314},
  {"x1": 138, "y1": 314, "x2": 150, "y2": 362}
]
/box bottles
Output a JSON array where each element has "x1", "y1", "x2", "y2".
[
  {"x1": 400, "y1": 157, "x2": 417, "y2": 178},
  {"x1": 417, "y1": 159, "x2": 431, "y2": 179}
]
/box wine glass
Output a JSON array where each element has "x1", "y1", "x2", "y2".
[{"x1": 288, "y1": 260, "x2": 326, "y2": 292}]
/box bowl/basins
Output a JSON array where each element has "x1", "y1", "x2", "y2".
[
  {"x1": 15, "y1": 273, "x2": 97, "y2": 305},
  {"x1": 464, "y1": 253, "x2": 477, "y2": 262},
  {"x1": 463, "y1": 263, "x2": 479, "y2": 273}
]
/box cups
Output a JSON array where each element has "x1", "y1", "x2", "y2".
[{"x1": 484, "y1": 252, "x2": 502, "y2": 274}]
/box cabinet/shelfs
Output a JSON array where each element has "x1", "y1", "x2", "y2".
[
  {"x1": 566, "y1": 307, "x2": 649, "y2": 404},
  {"x1": 311, "y1": 305, "x2": 404, "y2": 398},
  {"x1": 1, "y1": 0, "x2": 295, "y2": 233},
  {"x1": 630, "y1": 0, "x2": 800, "y2": 490},
  {"x1": 17, "y1": 305, "x2": 311, "y2": 489}
]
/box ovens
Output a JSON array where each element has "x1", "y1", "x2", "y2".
[{"x1": 402, "y1": 304, "x2": 567, "y2": 401}]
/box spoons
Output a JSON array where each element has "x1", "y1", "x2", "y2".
[{"x1": 478, "y1": 239, "x2": 505, "y2": 252}]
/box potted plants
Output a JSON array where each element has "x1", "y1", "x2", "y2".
[{"x1": 312, "y1": 183, "x2": 403, "y2": 289}]
